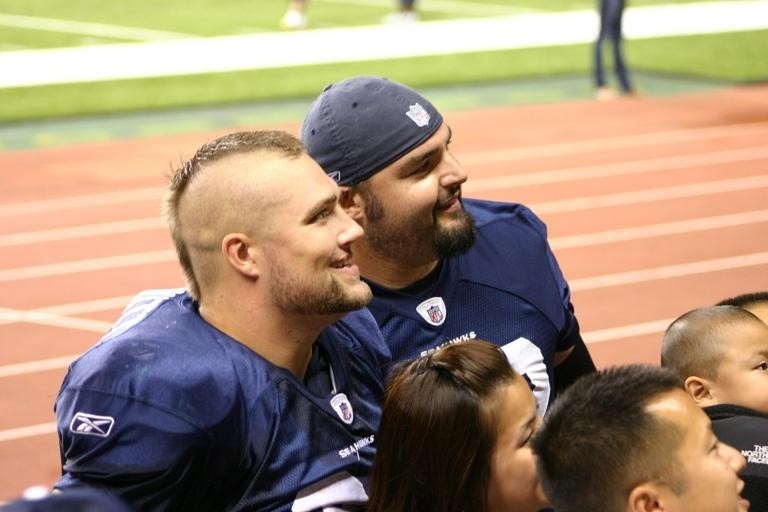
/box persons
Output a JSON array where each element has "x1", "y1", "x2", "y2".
[
  {"x1": 49, "y1": 127, "x2": 396, "y2": 511},
  {"x1": 712, "y1": 291, "x2": 767, "y2": 328},
  {"x1": 351, "y1": 336, "x2": 562, "y2": 510},
  {"x1": 521, "y1": 361, "x2": 754, "y2": 512},
  {"x1": 381, "y1": 0, "x2": 422, "y2": 28},
  {"x1": 280, "y1": 0, "x2": 312, "y2": 27},
  {"x1": 659, "y1": 304, "x2": 767, "y2": 512},
  {"x1": 294, "y1": 75, "x2": 602, "y2": 490},
  {"x1": 590, "y1": 0, "x2": 642, "y2": 103}
]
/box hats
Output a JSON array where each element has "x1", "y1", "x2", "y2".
[{"x1": 298, "y1": 74, "x2": 443, "y2": 189}]
